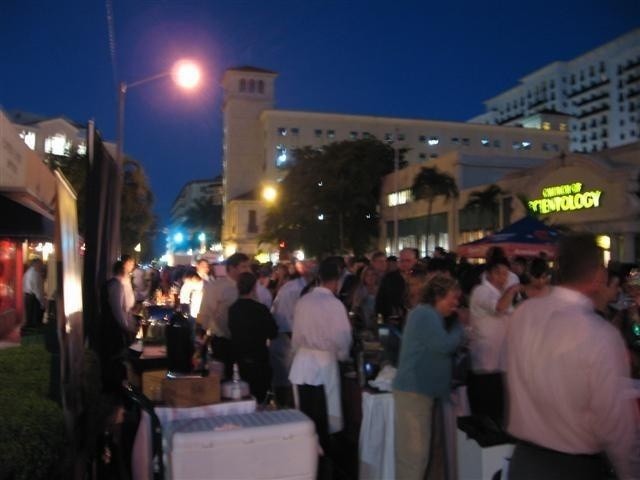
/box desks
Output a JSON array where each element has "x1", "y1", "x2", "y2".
[
  {"x1": 455, "y1": 414, "x2": 522, "y2": 480},
  {"x1": 153, "y1": 391, "x2": 257, "y2": 427},
  {"x1": 356, "y1": 378, "x2": 474, "y2": 480}
]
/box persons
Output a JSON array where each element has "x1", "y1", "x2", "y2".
[{"x1": 14, "y1": 233, "x2": 639, "y2": 479}]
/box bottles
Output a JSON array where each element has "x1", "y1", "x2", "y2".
[{"x1": 232, "y1": 362, "x2": 241, "y2": 400}]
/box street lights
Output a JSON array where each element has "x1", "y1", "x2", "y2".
[{"x1": 108, "y1": 57, "x2": 200, "y2": 292}]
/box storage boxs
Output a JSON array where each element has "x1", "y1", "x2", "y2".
[
  {"x1": 163, "y1": 409, "x2": 319, "y2": 479},
  {"x1": 161, "y1": 376, "x2": 222, "y2": 409},
  {"x1": 142, "y1": 369, "x2": 169, "y2": 402}
]
